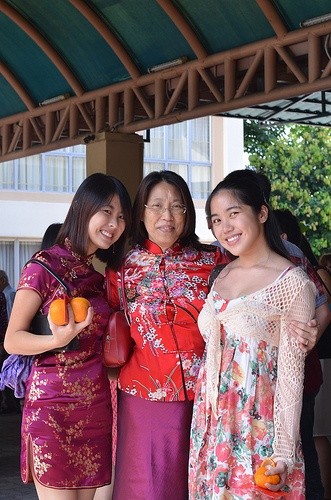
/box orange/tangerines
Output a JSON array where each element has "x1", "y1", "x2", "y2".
[
  {"x1": 69, "y1": 297, "x2": 90, "y2": 322},
  {"x1": 255, "y1": 458, "x2": 280, "y2": 488},
  {"x1": 50, "y1": 298, "x2": 68, "y2": 326}
]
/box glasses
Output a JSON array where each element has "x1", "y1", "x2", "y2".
[{"x1": 144, "y1": 204, "x2": 187, "y2": 216}]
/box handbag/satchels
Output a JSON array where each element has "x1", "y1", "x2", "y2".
[
  {"x1": 100, "y1": 265, "x2": 134, "y2": 369},
  {"x1": 22, "y1": 258, "x2": 81, "y2": 355}
]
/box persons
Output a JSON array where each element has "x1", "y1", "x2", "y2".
[
  {"x1": 0, "y1": 270, "x2": 18, "y2": 416},
  {"x1": 108, "y1": 168, "x2": 331, "y2": 500},
  {"x1": 185, "y1": 178, "x2": 317, "y2": 500},
  {"x1": 4, "y1": 171, "x2": 136, "y2": 500}
]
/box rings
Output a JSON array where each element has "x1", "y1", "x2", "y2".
[{"x1": 305, "y1": 340, "x2": 309, "y2": 345}]
